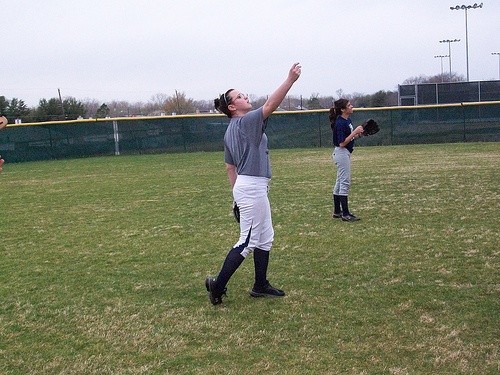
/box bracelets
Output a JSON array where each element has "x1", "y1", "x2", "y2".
[{"x1": 358, "y1": 133, "x2": 361, "y2": 139}]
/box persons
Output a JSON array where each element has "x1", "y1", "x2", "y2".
[
  {"x1": 205, "y1": 62, "x2": 302, "y2": 305},
  {"x1": 329, "y1": 98, "x2": 364, "y2": 221}
]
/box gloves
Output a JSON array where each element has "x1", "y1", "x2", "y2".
[
  {"x1": 232, "y1": 200, "x2": 240, "y2": 223},
  {"x1": 361, "y1": 118, "x2": 380, "y2": 136}
]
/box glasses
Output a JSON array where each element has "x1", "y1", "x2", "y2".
[{"x1": 231, "y1": 93, "x2": 248, "y2": 104}]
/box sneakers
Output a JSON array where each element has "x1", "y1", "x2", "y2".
[
  {"x1": 206, "y1": 278, "x2": 227, "y2": 305},
  {"x1": 250, "y1": 281, "x2": 285, "y2": 297},
  {"x1": 333, "y1": 210, "x2": 343, "y2": 218},
  {"x1": 341, "y1": 213, "x2": 360, "y2": 221}
]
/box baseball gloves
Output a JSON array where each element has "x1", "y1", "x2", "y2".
[
  {"x1": 362, "y1": 118, "x2": 380, "y2": 136},
  {"x1": 233, "y1": 204, "x2": 241, "y2": 223}
]
